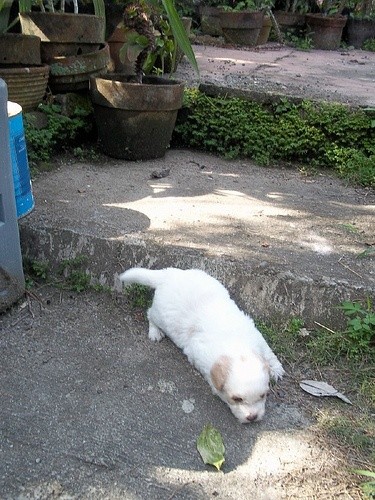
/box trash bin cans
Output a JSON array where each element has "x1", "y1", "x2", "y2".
[{"x1": 0, "y1": 77, "x2": 29, "y2": 313}]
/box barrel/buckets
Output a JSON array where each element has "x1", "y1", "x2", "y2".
[{"x1": 7, "y1": 101, "x2": 35, "y2": 219}]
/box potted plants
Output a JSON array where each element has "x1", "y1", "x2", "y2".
[
  {"x1": 88, "y1": 0, "x2": 200, "y2": 159},
  {"x1": 175, "y1": 0, "x2": 375, "y2": 50}
]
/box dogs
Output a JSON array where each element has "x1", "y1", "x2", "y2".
[{"x1": 118, "y1": 267, "x2": 285, "y2": 424}]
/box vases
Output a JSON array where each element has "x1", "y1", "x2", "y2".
[
  {"x1": 154, "y1": 16, "x2": 192, "y2": 74},
  {"x1": 105, "y1": 28, "x2": 144, "y2": 74},
  {"x1": 0, "y1": 64, "x2": 49, "y2": 113},
  {"x1": 0, "y1": 33, "x2": 41, "y2": 68},
  {"x1": 40, "y1": 41, "x2": 109, "y2": 99},
  {"x1": 18, "y1": 13, "x2": 106, "y2": 58}
]
